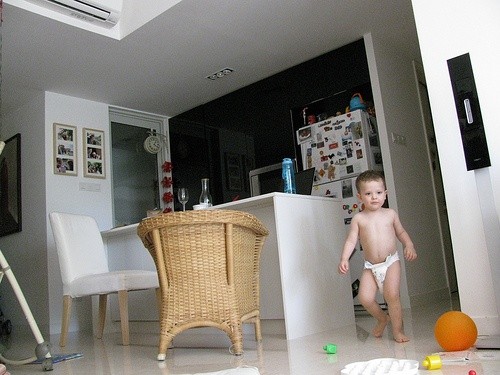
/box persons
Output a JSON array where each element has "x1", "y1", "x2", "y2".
[
  {"x1": 56, "y1": 130, "x2": 73, "y2": 171},
  {"x1": 88, "y1": 135, "x2": 102, "y2": 174},
  {"x1": 338, "y1": 170, "x2": 417, "y2": 342}
]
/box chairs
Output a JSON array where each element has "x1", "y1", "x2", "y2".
[
  {"x1": 49, "y1": 212, "x2": 161, "y2": 346},
  {"x1": 137, "y1": 210, "x2": 268, "y2": 361}
]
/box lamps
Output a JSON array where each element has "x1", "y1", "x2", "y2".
[{"x1": 44, "y1": 0, "x2": 121, "y2": 27}]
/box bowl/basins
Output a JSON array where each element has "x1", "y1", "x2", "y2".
[
  {"x1": 192, "y1": 204, "x2": 208, "y2": 210},
  {"x1": 146, "y1": 208, "x2": 163, "y2": 216}
]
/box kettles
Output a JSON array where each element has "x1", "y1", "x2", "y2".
[{"x1": 349, "y1": 92, "x2": 367, "y2": 111}]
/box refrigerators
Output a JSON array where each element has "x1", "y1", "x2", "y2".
[{"x1": 295, "y1": 109, "x2": 389, "y2": 316}]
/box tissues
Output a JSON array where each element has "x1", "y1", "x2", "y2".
[{"x1": 193, "y1": 199, "x2": 212, "y2": 210}]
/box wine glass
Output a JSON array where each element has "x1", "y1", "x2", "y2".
[{"x1": 177, "y1": 188, "x2": 189, "y2": 211}]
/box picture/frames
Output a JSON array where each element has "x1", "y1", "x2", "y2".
[
  {"x1": 225, "y1": 151, "x2": 242, "y2": 191},
  {"x1": 53, "y1": 123, "x2": 78, "y2": 176},
  {"x1": 82, "y1": 128, "x2": 106, "y2": 179},
  {"x1": 0, "y1": 133, "x2": 22, "y2": 237}
]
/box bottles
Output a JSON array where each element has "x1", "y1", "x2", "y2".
[
  {"x1": 199, "y1": 179, "x2": 212, "y2": 205},
  {"x1": 282, "y1": 158, "x2": 296, "y2": 194},
  {"x1": 300, "y1": 107, "x2": 308, "y2": 127},
  {"x1": 421, "y1": 348, "x2": 481, "y2": 370}
]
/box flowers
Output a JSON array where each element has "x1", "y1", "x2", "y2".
[
  {"x1": 161, "y1": 161, "x2": 174, "y2": 172},
  {"x1": 163, "y1": 192, "x2": 175, "y2": 203},
  {"x1": 161, "y1": 176, "x2": 174, "y2": 186},
  {"x1": 163, "y1": 208, "x2": 172, "y2": 212}
]
